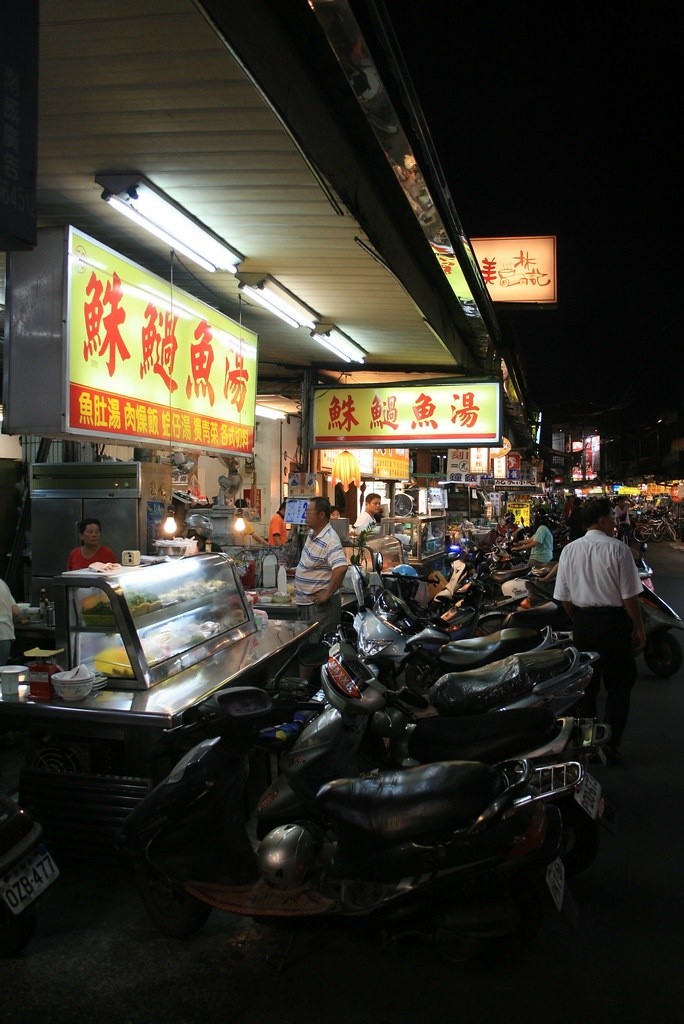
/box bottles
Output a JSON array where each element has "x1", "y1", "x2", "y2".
[
  {"x1": 277, "y1": 565, "x2": 287, "y2": 593},
  {"x1": 46, "y1": 602, "x2": 56, "y2": 627},
  {"x1": 40, "y1": 589, "x2": 48, "y2": 618},
  {"x1": 262, "y1": 550, "x2": 277, "y2": 587}
]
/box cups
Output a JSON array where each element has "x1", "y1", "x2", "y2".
[{"x1": 1, "y1": 673, "x2": 18, "y2": 696}]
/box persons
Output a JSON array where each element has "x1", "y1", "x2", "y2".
[
  {"x1": 553, "y1": 498, "x2": 646, "y2": 767},
  {"x1": 542, "y1": 497, "x2": 546, "y2": 505},
  {"x1": 269, "y1": 501, "x2": 288, "y2": 546},
  {"x1": 0, "y1": 578, "x2": 19, "y2": 666},
  {"x1": 503, "y1": 512, "x2": 518, "y2": 533},
  {"x1": 563, "y1": 495, "x2": 591, "y2": 541},
  {"x1": 66, "y1": 518, "x2": 117, "y2": 569},
  {"x1": 230, "y1": 499, "x2": 269, "y2": 546},
  {"x1": 330, "y1": 505, "x2": 341, "y2": 517},
  {"x1": 353, "y1": 494, "x2": 381, "y2": 533},
  {"x1": 511, "y1": 508, "x2": 554, "y2": 567},
  {"x1": 613, "y1": 495, "x2": 635, "y2": 547},
  {"x1": 294, "y1": 497, "x2": 348, "y2": 703},
  {"x1": 184, "y1": 514, "x2": 224, "y2": 553}
]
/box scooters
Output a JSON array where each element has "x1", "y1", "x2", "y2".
[
  {"x1": 1, "y1": 792, "x2": 61, "y2": 959},
  {"x1": 633, "y1": 542, "x2": 684, "y2": 679},
  {"x1": 119, "y1": 512, "x2": 633, "y2": 1008}
]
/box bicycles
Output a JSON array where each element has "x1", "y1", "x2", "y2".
[{"x1": 633, "y1": 510, "x2": 677, "y2": 543}]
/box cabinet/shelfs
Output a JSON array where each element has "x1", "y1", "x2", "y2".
[{"x1": 54, "y1": 551, "x2": 258, "y2": 691}]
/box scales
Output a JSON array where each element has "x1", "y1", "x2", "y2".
[{"x1": 25, "y1": 647, "x2": 65, "y2": 700}]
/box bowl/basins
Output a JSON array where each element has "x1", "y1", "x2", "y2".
[
  {"x1": 51, "y1": 672, "x2": 95, "y2": 701},
  {"x1": 156, "y1": 547, "x2": 186, "y2": 556}
]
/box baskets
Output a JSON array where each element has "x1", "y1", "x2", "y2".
[{"x1": 383, "y1": 576, "x2": 420, "y2": 599}]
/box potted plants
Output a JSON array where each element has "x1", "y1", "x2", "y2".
[{"x1": 342, "y1": 522, "x2": 377, "y2": 591}]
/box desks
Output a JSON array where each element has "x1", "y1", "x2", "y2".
[
  {"x1": 0, "y1": 620, "x2": 322, "y2": 855},
  {"x1": 6, "y1": 621, "x2": 54, "y2": 663},
  {"x1": 249, "y1": 588, "x2": 358, "y2": 620}
]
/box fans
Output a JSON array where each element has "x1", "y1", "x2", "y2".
[
  {"x1": 157, "y1": 452, "x2": 199, "y2": 476},
  {"x1": 396, "y1": 493, "x2": 415, "y2": 516},
  {"x1": 212, "y1": 472, "x2": 244, "y2": 511}
]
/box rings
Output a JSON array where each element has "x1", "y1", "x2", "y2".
[{"x1": 316, "y1": 600, "x2": 318, "y2": 602}]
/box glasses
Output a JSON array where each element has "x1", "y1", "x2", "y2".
[{"x1": 305, "y1": 510, "x2": 321, "y2": 516}]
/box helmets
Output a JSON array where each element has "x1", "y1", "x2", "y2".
[
  {"x1": 504, "y1": 512, "x2": 515, "y2": 523},
  {"x1": 256, "y1": 824, "x2": 318, "y2": 890},
  {"x1": 391, "y1": 564, "x2": 418, "y2": 578},
  {"x1": 371, "y1": 705, "x2": 407, "y2": 739},
  {"x1": 182, "y1": 513, "x2": 213, "y2": 538},
  {"x1": 534, "y1": 508, "x2": 548, "y2": 525}
]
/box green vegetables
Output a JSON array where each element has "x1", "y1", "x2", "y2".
[{"x1": 82, "y1": 591, "x2": 158, "y2": 615}]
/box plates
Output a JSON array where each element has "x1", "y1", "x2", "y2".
[{"x1": 92, "y1": 676, "x2": 108, "y2": 691}]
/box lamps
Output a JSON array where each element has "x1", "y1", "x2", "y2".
[
  {"x1": 256, "y1": 404, "x2": 288, "y2": 420},
  {"x1": 95, "y1": 172, "x2": 245, "y2": 276},
  {"x1": 331, "y1": 449, "x2": 360, "y2": 492},
  {"x1": 310, "y1": 324, "x2": 367, "y2": 364},
  {"x1": 232, "y1": 272, "x2": 321, "y2": 330}
]
